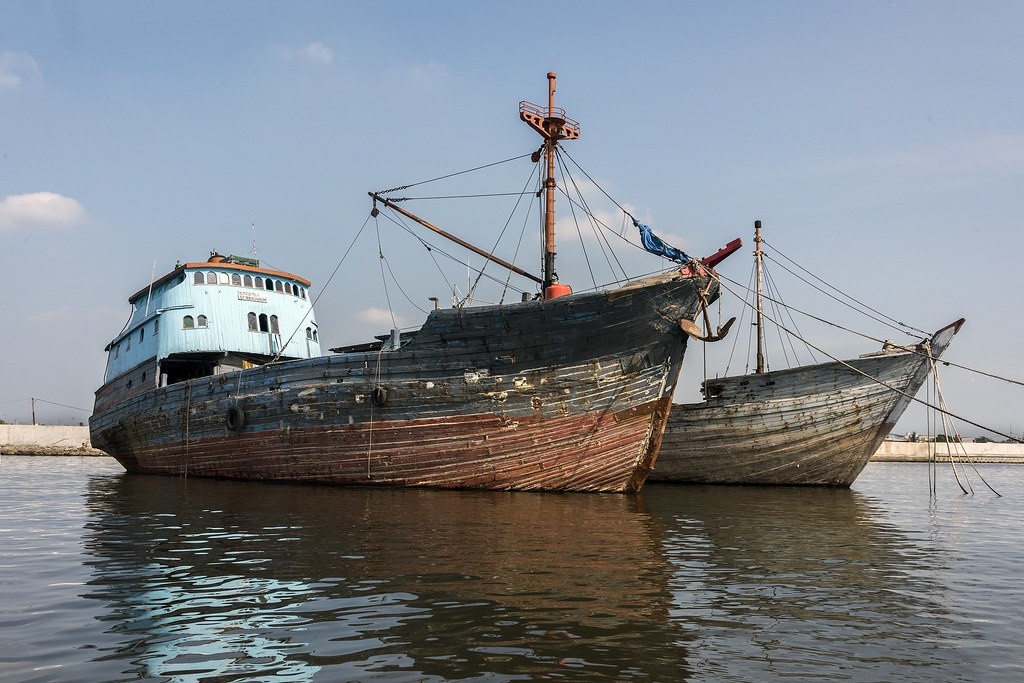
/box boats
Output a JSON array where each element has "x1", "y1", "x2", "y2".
[
  {"x1": 86, "y1": 71, "x2": 744, "y2": 497},
  {"x1": 644, "y1": 220, "x2": 966, "y2": 489}
]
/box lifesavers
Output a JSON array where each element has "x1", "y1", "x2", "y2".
[
  {"x1": 371, "y1": 388, "x2": 387, "y2": 404},
  {"x1": 226, "y1": 406, "x2": 245, "y2": 429}
]
